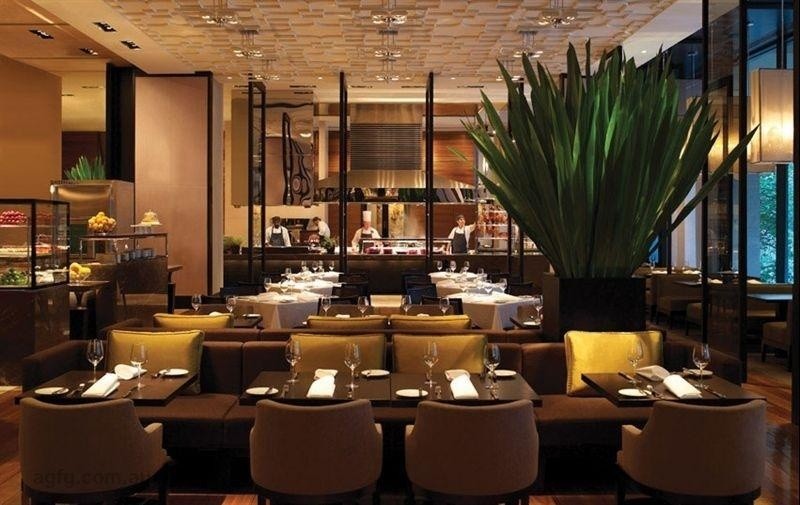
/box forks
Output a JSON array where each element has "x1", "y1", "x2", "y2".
[
  {"x1": 434, "y1": 384, "x2": 442, "y2": 399},
  {"x1": 65, "y1": 383, "x2": 84, "y2": 399},
  {"x1": 279, "y1": 385, "x2": 290, "y2": 399}
]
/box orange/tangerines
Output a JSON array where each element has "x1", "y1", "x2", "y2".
[{"x1": 0, "y1": 269, "x2": 28, "y2": 285}]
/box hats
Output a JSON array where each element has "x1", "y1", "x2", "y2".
[{"x1": 362, "y1": 211, "x2": 372, "y2": 222}]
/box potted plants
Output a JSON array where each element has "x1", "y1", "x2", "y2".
[{"x1": 445, "y1": 36, "x2": 761, "y2": 337}]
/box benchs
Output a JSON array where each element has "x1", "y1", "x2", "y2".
[{"x1": 22, "y1": 329, "x2": 744, "y2": 448}]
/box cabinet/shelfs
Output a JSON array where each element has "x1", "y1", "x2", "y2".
[
  {"x1": 0, "y1": 197, "x2": 72, "y2": 291},
  {"x1": 79, "y1": 232, "x2": 170, "y2": 268}
]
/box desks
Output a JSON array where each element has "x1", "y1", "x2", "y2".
[{"x1": 165, "y1": 262, "x2": 184, "y2": 313}]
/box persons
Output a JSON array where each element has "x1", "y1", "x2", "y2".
[
  {"x1": 265, "y1": 216, "x2": 292, "y2": 247},
  {"x1": 443, "y1": 214, "x2": 477, "y2": 254},
  {"x1": 312, "y1": 217, "x2": 330, "y2": 246},
  {"x1": 351, "y1": 220, "x2": 384, "y2": 254}
]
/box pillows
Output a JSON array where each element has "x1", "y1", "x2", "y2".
[
  {"x1": 291, "y1": 334, "x2": 385, "y2": 376},
  {"x1": 108, "y1": 330, "x2": 203, "y2": 396},
  {"x1": 392, "y1": 332, "x2": 487, "y2": 379},
  {"x1": 565, "y1": 329, "x2": 665, "y2": 396}
]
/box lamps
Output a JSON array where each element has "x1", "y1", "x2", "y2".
[
  {"x1": 496, "y1": 2, "x2": 576, "y2": 82},
  {"x1": 199, "y1": 0, "x2": 581, "y2": 86},
  {"x1": 200, "y1": 0, "x2": 279, "y2": 82},
  {"x1": 371, "y1": 0, "x2": 408, "y2": 83},
  {"x1": 750, "y1": 66, "x2": 797, "y2": 167}
]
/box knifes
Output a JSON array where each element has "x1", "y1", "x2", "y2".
[
  {"x1": 618, "y1": 371, "x2": 641, "y2": 383},
  {"x1": 50, "y1": 383, "x2": 71, "y2": 393},
  {"x1": 266, "y1": 386, "x2": 272, "y2": 395},
  {"x1": 418, "y1": 386, "x2": 423, "y2": 397},
  {"x1": 704, "y1": 387, "x2": 727, "y2": 399},
  {"x1": 345, "y1": 388, "x2": 352, "y2": 399}
]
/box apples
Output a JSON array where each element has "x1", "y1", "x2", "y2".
[
  {"x1": 69, "y1": 262, "x2": 91, "y2": 278},
  {"x1": 0, "y1": 210, "x2": 28, "y2": 225}
]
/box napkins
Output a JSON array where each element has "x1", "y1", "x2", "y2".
[
  {"x1": 82, "y1": 374, "x2": 121, "y2": 399},
  {"x1": 307, "y1": 375, "x2": 336, "y2": 399},
  {"x1": 663, "y1": 375, "x2": 702, "y2": 401},
  {"x1": 450, "y1": 376, "x2": 479, "y2": 399}
]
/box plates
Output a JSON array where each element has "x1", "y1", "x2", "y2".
[
  {"x1": 681, "y1": 367, "x2": 711, "y2": 377},
  {"x1": 618, "y1": 387, "x2": 649, "y2": 398},
  {"x1": 245, "y1": 386, "x2": 277, "y2": 395},
  {"x1": 159, "y1": 368, "x2": 187, "y2": 376},
  {"x1": 34, "y1": 386, "x2": 68, "y2": 397},
  {"x1": 488, "y1": 367, "x2": 517, "y2": 377},
  {"x1": 393, "y1": 388, "x2": 427, "y2": 398},
  {"x1": 359, "y1": 368, "x2": 389, "y2": 378}
]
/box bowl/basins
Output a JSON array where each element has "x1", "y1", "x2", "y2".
[{"x1": 70, "y1": 272, "x2": 90, "y2": 286}]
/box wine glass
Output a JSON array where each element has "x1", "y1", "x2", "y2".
[
  {"x1": 284, "y1": 337, "x2": 301, "y2": 384},
  {"x1": 343, "y1": 341, "x2": 366, "y2": 390},
  {"x1": 86, "y1": 338, "x2": 105, "y2": 384},
  {"x1": 401, "y1": 258, "x2": 543, "y2": 322},
  {"x1": 422, "y1": 339, "x2": 439, "y2": 385},
  {"x1": 129, "y1": 342, "x2": 150, "y2": 391},
  {"x1": 189, "y1": 259, "x2": 373, "y2": 321},
  {"x1": 691, "y1": 342, "x2": 712, "y2": 389},
  {"x1": 627, "y1": 338, "x2": 643, "y2": 384},
  {"x1": 481, "y1": 344, "x2": 503, "y2": 391}
]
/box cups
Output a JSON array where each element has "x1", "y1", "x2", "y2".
[{"x1": 120, "y1": 248, "x2": 155, "y2": 262}]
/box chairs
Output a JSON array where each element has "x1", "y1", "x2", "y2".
[
  {"x1": 617, "y1": 399, "x2": 764, "y2": 505},
  {"x1": 407, "y1": 398, "x2": 540, "y2": 505},
  {"x1": 250, "y1": 399, "x2": 383, "y2": 505},
  {"x1": 108, "y1": 277, "x2": 553, "y2": 343},
  {"x1": 18, "y1": 395, "x2": 181, "y2": 505}
]
